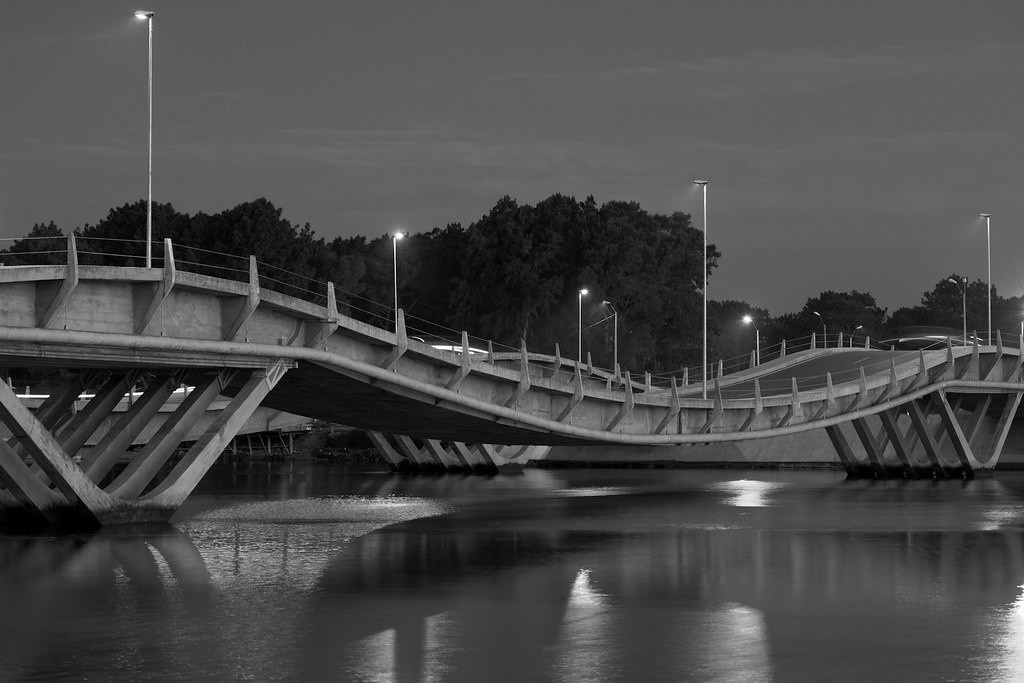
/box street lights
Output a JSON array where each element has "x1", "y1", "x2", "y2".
[
  {"x1": 603, "y1": 301, "x2": 618, "y2": 375},
  {"x1": 579, "y1": 289, "x2": 588, "y2": 363},
  {"x1": 394, "y1": 234, "x2": 404, "y2": 337},
  {"x1": 744, "y1": 317, "x2": 759, "y2": 366},
  {"x1": 814, "y1": 312, "x2": 826, "y2": 348},
  {"x1": 694, "y1": 180, "x2": 708, "y2": 399},
  {"x1": 948, "y1": 278, "x2": 966, "y2": 346},
  {"x1": 852, "y1": 326, "x2": 863, "y2": 337},
  {"x1": 133, "y1": 11, "x2": 154, "y2": 270},
  {"x1": 979, "y1": 214, "x2": 991, "y2": 345}
]
